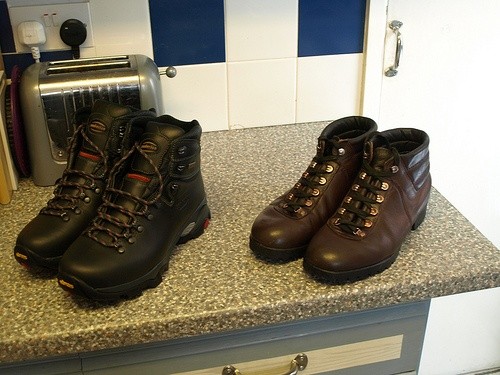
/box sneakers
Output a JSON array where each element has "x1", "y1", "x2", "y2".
[
  {"x1": 14, "y1": 100, "x2": 158, "y2": 275},
  {"x1": 58, "y1": 114, "x2": 211, "y2": 302}
]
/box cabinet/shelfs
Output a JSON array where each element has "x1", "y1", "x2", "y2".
[{"x1": 0, "y1": 120, "x2": 500, "y2": 375}]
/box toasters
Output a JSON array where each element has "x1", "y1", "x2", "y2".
[{"x1": 21, "y1": 54, "x2": 176, "y2": 187}]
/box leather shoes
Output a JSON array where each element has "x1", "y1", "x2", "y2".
[
  {"x1": 303, "y1": 128, "x2": 431, "y2": 285},
  {"x1": 249, "y1": 115, "x2": 378, "y2": 264}
]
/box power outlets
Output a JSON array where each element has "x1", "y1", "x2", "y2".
[{"x1": 8, "y1": 2, "x2": 95, "y2": 53}]
